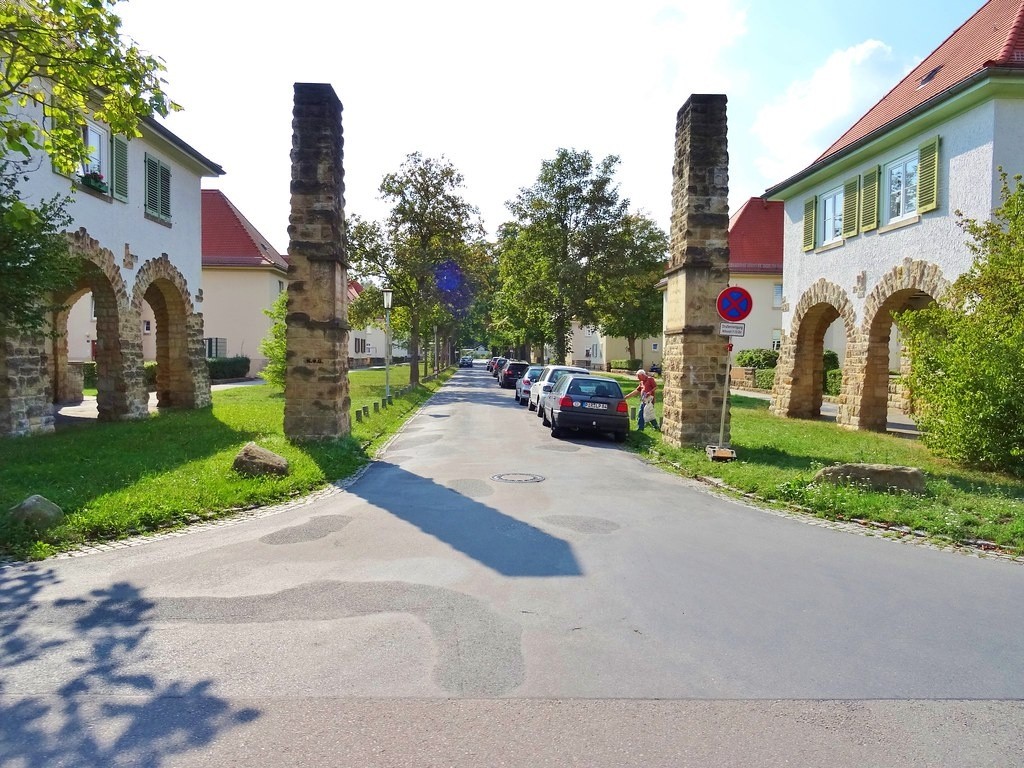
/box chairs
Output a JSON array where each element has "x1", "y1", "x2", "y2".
[{"x1": 594, "y1": 385, "x2": 606, "y2": 395}]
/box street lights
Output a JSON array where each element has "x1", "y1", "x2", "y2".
[
  {"x1": 433, "y1": 322, "x2": 439, "y2": 374},
  {"x1": 382, "y1": 289, "x2": 393, "y2": 396}
]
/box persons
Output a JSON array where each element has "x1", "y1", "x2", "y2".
[
  {"x1": 624, "y1": 369, "x2": 660, "y2": 432},
  {"x1": 649, "y1": 364, "x2": 657, "y2": 372}
]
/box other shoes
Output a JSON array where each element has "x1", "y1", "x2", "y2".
[
  {"x1": 637, "y1": 429, "x2": 645, "y2": 431},
  {"x1": 654, "y1": 427, "x2": 661, "y2": 431}
]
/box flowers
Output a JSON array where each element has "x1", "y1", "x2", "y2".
[{"x1": 87, "y1": 172, "x2": 104, "y2": 180}]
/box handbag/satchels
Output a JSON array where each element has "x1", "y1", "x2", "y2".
[{"x1": 643, "y1": 398, "x2": 656, "y2": 423}]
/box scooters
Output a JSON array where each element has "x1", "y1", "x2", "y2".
[{"x1": 650, "y1": 362, "x2": 661, "y2": 374}]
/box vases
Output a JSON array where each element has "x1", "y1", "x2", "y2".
[{"x1": 81, "y1": 174, "x2": 108, "y2": 194}]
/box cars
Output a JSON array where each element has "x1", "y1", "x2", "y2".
[
  {"x1": 497, "y1": 359, "x2": 529, "y2": 388},
  {"x1": 542, "y1": 374, "x2": 630, "y2": 441},
  {"x1": 492, "y1": 359, "x2": 507, "y2": 377},
  {"x1": 459, "y1": 356, "x2": 472, "y2": 368},
  {"x1": 515, "y1": 365, "x2": 545, "y2": 405},
  {"x1": 528, "y1": 365, "x2": 590, "y2": 417},
  {"x1": 486, "y1": 357, "x2": 501, "y2": 372}
]
